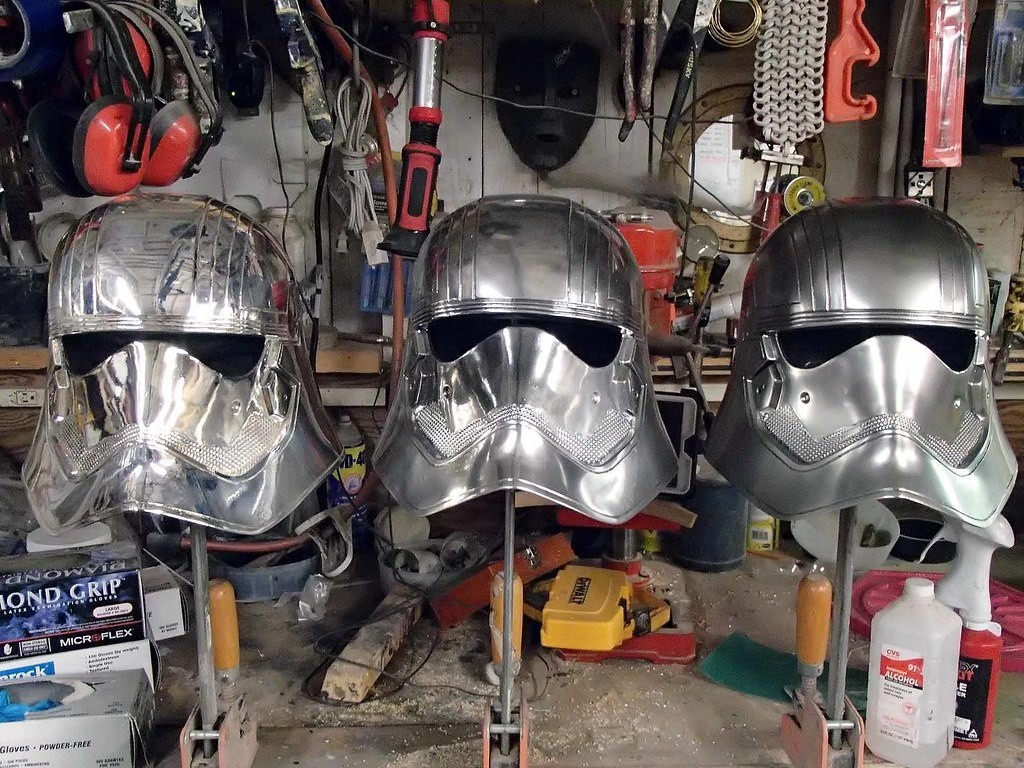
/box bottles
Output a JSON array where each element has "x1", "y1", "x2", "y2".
[
  {"x1": 953, "y1": 620, "x2": 1004, "y2": 750},
  {"x1": 261, "y1": 206, "x2": 306, "y2": 282},
  {"x1": 747, "y1": 501, "x2": 780, "y2": 552},
  {"x1": 368, "y1": 150, "x2": 402, "y2": 233},
  {"x1": 864, "y1": 577, "x2": 962, "y2": 768},
  {"x1": 327, "y1": 416, "x2": 367, "y2": 524}
]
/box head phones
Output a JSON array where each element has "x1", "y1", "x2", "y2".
[{"x1": 25, "y1": 0, "x2": 226, "y2": 196}]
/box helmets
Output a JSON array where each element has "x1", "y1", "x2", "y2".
[
  {"x1": 372, "y1": 193, "x2": 680, "y2": 524},
  {"x1": 23, "y1": 193, "x2": 346, "y2": 536},
  {"x1": 702, "y1": 197, "x2": 1018, "y2": 529}
]
